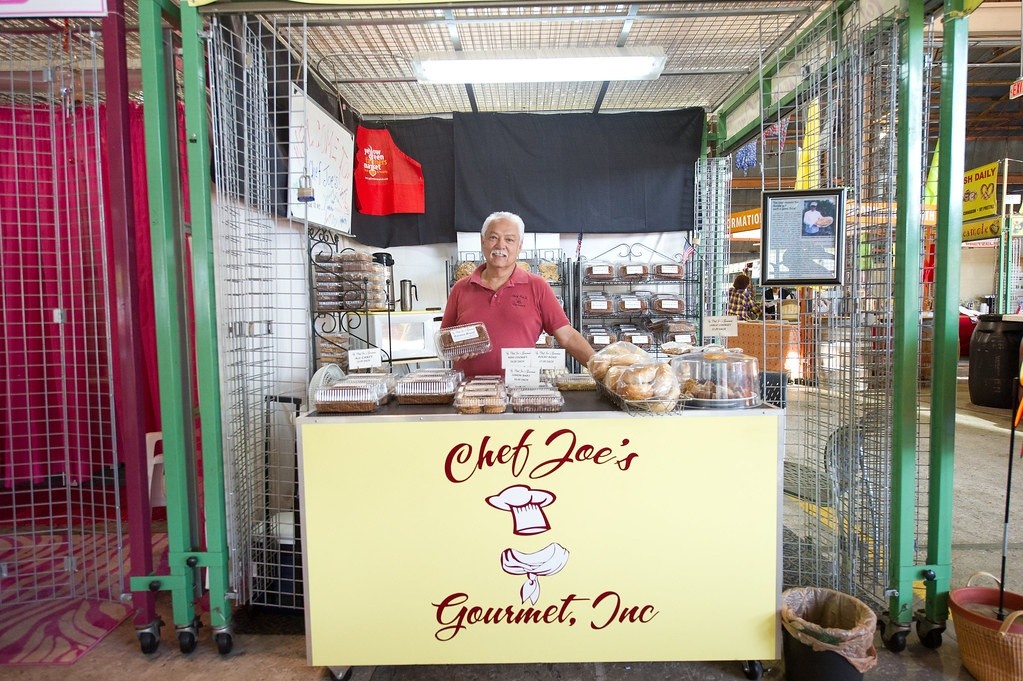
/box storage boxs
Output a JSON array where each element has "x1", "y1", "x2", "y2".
[{"x1": 311, "y1": 366, "x2": 596, "y2": 414}]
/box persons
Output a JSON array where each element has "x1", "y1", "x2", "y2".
[
  {"x1": 765, "y1": 287, "x2": 792, "y2": 320},
  {"x1": 439, "y1": 212, "x2": 596, "y2": 377},
  {"x1": 729, "y1": 275, "x2": 762, "y2": 322}
]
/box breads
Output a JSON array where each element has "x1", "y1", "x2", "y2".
[
  {"x1": 589, "y1": 345, "x2": 681, "y2": 414},
  {"x1": 314, "y1": 367, "x2": 464, "y2": 413},
  {"x1": 440, "y1": 325, "x2": 490, "y2": 356},
  {"x1": 458, "y1": 380, "x2": 563, "y2": 414}
]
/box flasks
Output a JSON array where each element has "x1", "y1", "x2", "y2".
[{"x1": 373, "y1": 252, "x2": 395, "y2": 311}]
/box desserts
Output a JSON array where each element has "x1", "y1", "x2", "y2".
[{"x1": 682, "y1": 378, "x2": 743, "y2": 400}]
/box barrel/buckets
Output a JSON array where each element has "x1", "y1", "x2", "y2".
[{"x1": 969, "y1": 312, "x2": 1023, "y2": 409}]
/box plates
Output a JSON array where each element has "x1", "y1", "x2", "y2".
[{"x1": 691, "y1": 390, "x2": 757, "y2": 408}]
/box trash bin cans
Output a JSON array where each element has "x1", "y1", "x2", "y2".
[
  {"x1": 760, "y1": 370, "x2": 787, "y2": 409},
  {"x1": 781, "y1": 585, "x2": 878, "y2": 681}
]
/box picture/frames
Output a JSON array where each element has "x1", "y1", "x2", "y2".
[{"x1": 760, "y1": 188, "x2": 845, "y2": 286}]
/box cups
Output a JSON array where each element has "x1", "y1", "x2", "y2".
[{"x1": 400, "y1": 279, "x2": 419, "y2": 312}]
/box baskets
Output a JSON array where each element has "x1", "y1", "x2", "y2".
[{"x1": 949, "y1": 571, "x2": 1023, "y2": 681}]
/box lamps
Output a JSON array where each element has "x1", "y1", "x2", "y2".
[{"x1": 413, "y1": 44, "x2": 669, "y2": 84}]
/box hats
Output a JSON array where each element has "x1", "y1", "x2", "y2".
[{"x1": 810, "y1": 202, "x2": 817, "y2": 206}]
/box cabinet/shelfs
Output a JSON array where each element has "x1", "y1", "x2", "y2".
[
  {"x1": 442, "y1": 248, "x2": 577, "y2": 373},
  {"x1": 574, "y1": 241, "x2": 703, "y2": 374},
  {"x1": 306, "y1": 252, "x2": 395, "y2": 374}
]
